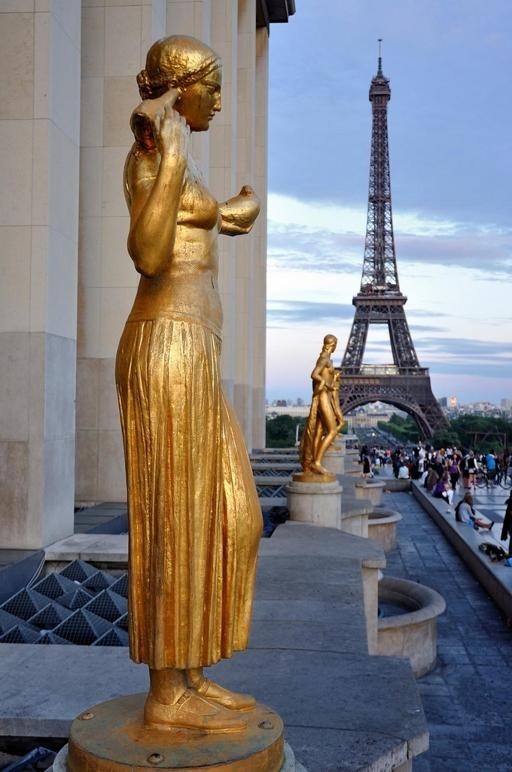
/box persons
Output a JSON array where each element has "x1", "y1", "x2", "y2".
[
  {"x1": 358, "y1": 441, "x2": 512, "y2": 560},
  {"x1": 298, "y1": 334, "x2": 345, "y2": 475},
  {"x1": 114, "y1": 36, "x2": 264, "y2": 734}
]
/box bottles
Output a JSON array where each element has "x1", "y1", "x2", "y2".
[{"x1": 466, "y1": 519, "x2": 473, "y2": 537}]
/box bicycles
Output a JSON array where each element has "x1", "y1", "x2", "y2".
[{"x1": 473, "y1": 465, "x2": 512, "y2": 490}]
